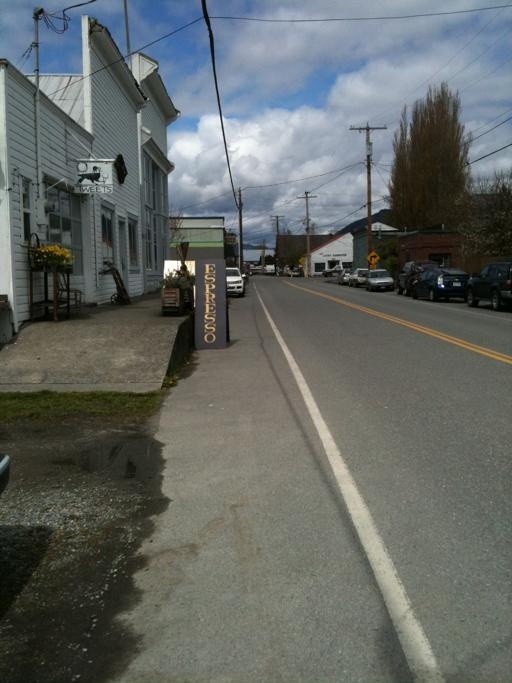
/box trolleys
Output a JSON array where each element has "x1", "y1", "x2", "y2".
[{"x1": 98, "y1": 261, "x2": 131, "y2": 305}]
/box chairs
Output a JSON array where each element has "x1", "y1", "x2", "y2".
[{"x1": 57, "y1": 271, "x2": 82, "y2": 314}]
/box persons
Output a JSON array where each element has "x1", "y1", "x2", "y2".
[{"x1": 288, "y1": 264, "x2": 296, "y2": 281}]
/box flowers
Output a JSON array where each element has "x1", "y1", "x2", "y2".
[{"x1": 36, "y1": 245, "x2": 74, "y2": 267}]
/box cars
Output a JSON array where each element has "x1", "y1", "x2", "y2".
[
  {"x1": 397, "y1": 260, "x2": 512, "y2": 312},
  {"x1": 322, "y1": 264, "x2": 395, "y2": 292},
  {"x1": 226, "y1": 267, "x2": 247, "y2": 297},
  {"x1": 248, "y1": 264, "x2": 300, "y2": 277}
]
